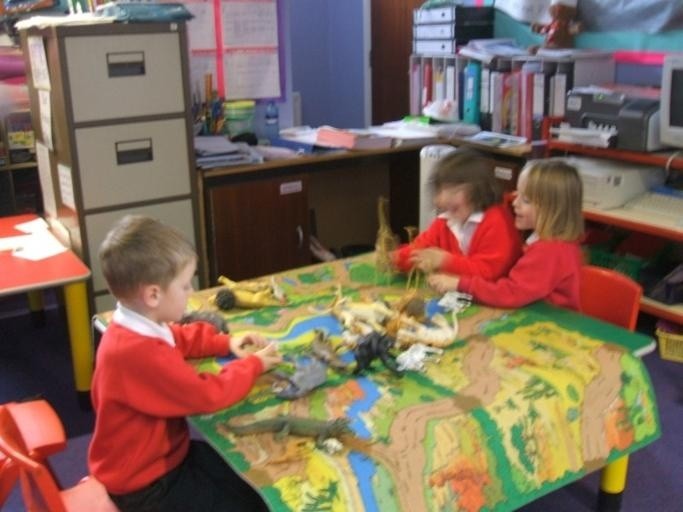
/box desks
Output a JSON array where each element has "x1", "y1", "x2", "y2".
[
  {"x1": 0, "y1": 212, "x2": 92, "y2": 410},
  {"x1": 91, "y1": 250, "x2": 656, "y2": 511},
  {"x1": 202, "y1": 139, "x2": 450, "y2": 297}
]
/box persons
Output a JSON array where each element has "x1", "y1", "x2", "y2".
[
  {"x1": 86, "y1": 213, "x2": 286, "y2": 512},
  {"x1": 392, "y1": 146, "x2": 524, "y2": 281},
  {"x1": 427, "y1": 157, "x2": 586, "y2": 313}
]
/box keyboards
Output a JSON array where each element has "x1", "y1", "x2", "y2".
[{"x1": 625, "y1": 191, "x2": 683, "y2": 220}]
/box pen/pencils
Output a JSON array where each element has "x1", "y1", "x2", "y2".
[{"x1": 192, "y1": 74, "x2": 227, "y2": 135}]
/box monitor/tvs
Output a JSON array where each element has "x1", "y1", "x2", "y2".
[{"x1": 660, "y1": 56, "x2": 683, "y2": 150}]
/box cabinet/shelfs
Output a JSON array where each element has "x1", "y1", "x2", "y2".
[
  {"x1": 21, "y1": 15, "x2": 202, "y2": 347},
  {"x1": 439, "y1": 117, "x2": 682, "y2": 322},
  {"x1": 0, "y1": 108, "x2": 38, "y2": 214}
]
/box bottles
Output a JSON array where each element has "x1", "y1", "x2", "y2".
[{"x1": 265, "y1": 101, "x2": 280, "y2": 141}]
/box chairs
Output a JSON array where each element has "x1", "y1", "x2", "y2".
[
  {"x1": 0, "y1": 399, "x2": 118, "y2": 512},
  {"x1": 580, "y1": 263, "x2": 644, "y2": 331}
]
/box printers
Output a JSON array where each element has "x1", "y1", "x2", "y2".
[{"x1": 565, "y1": 94, "x2": 670, "y2": 153}]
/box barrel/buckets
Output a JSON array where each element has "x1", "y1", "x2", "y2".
[{"x1": 222, "y1": 99, "x2": 257, "y2": 135}]
